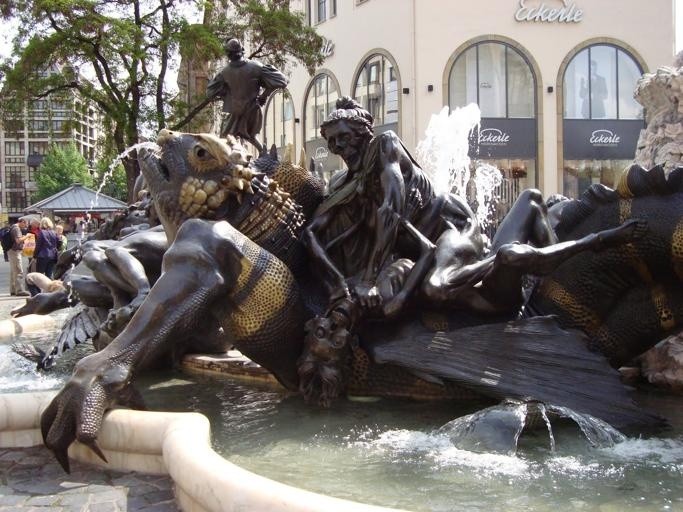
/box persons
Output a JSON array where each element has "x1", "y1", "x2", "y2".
[
  {"x1": 1, "y1": 215, "x2": 104, "y2": 298},
  {"x1": 206, "y1": 39, "x2": 286, "y2": 159},
  {"x1": 298, "y1": 95, "x2": 647, "y2": 410}
]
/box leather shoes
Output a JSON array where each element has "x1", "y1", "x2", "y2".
[{"x1": 10, "y1": 292, "x2": 30, "y2": 296}]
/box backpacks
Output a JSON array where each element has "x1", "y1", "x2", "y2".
[{"x1": 0, "y1": 225, "x2": 20, "y2": 252}]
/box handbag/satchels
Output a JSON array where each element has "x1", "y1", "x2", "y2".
[{"x1": 22, "y1": 232, "x2": 36, "y2": 257}]
